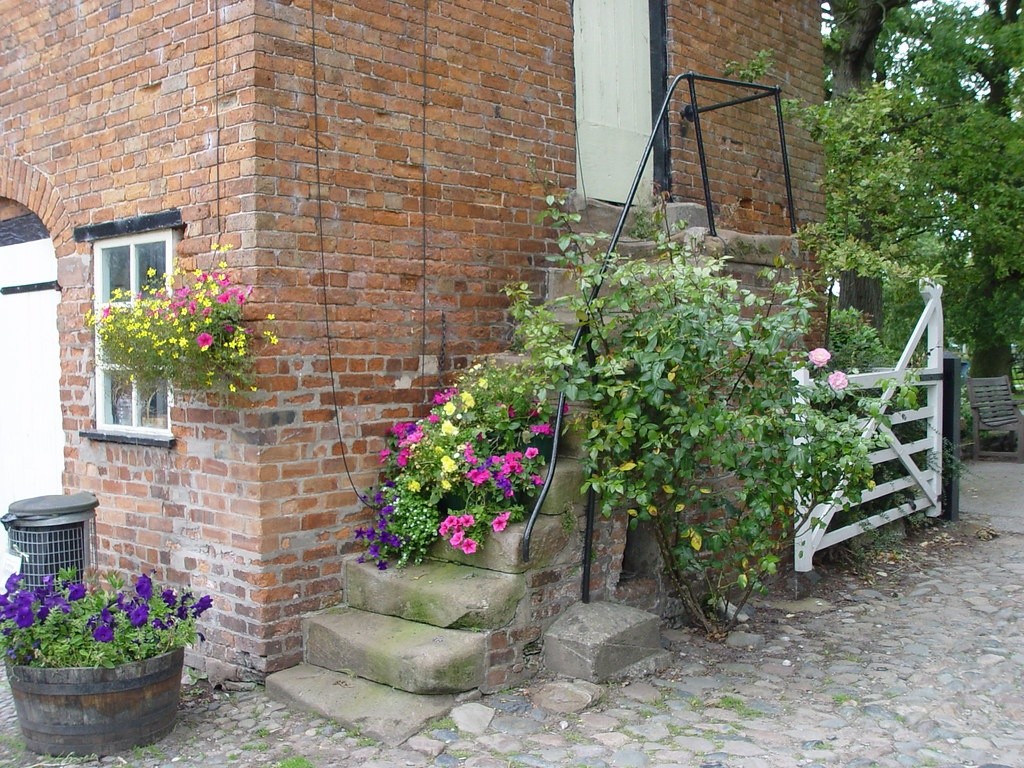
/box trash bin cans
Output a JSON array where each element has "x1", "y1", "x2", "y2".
[{"x1": 6, "y1": 490, "x2": 101, "y2": 594}]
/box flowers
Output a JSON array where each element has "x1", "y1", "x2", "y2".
[
  {"x1": 0, "y1": 565, "x2": 214, "y2": 669},
  {"x1": 83, "y1": 243, "x2": 279, "y2": 413},
  {"x1": 354, "y1": 353, "x2": 571, "y2": 571}
]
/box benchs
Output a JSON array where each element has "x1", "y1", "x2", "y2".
[{"x1": 967, "y1": 375, "x2": 1024, "y2": 464}]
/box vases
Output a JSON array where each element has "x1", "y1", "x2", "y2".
[{"x1": 5, "y1": 647, "x2": 183, "y2": 759}]
